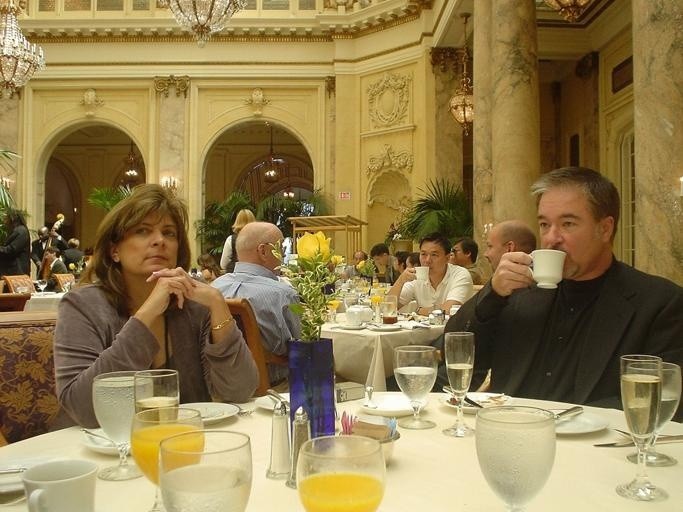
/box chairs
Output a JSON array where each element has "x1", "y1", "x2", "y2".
[{"x1": 0, "y1": 254, "x2": 94, "y2": 314}]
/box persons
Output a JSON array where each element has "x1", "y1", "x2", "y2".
[
  {"x1": 49, "y1": 182, "x2": 260, "y2": 432},
  {"x1": 1, "y1": 208, "x2": 86, "y2": 292},
  {"x1": 440, "y1": 165, "x2": 682, "y2": 424},
  {"x1": 484, "y1": 218, "x2": 536, "y2": 275},
  {"x1": 341, "y1": 237, "x2": 482, "y2": 290},
  {"x1": 197, "y1": 252, "x2": 222, "y2": 280},
  {"x1": 383, "y1": 231, "x2": 474, "y2": 320},
  {"x1": 220, "y1": 209, "x2": 257, "y2": 274},
  {"x1": 208, "y1": 221, "x2": 313, "y2": 393}
]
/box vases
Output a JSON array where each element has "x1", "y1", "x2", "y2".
[{"x1": 286, "y1": 337, "x2": 334, "y2": 441}]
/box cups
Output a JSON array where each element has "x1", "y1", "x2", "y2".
[
  {"x1": 21, "y1": 458, "x2": 99, "y2": 512},
  {"x1": 296, "y1": 435, "x2": 388, "y2": 511},
  {"x1": 524, "y1": 249, "x2": 567, "y2": 290},
  {"x1": 415, "y1": 266, "x2": 430, "y2": 283},
  {"x1": 326, "y1": 274, "x2": 460, "y2": 324}
]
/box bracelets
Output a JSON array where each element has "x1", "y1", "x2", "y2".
[
  {"x1": 212, "y1": 316, "x2": 233, "y2": 330},
  {"x1": 415, "y1": 306, "x2": 423, "y2": 315}
]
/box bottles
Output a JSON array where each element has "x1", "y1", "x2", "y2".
[{"x1": 265, "y1": 403, "x2": 310, "y2": 487}]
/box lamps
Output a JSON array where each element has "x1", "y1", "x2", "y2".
[
  {"x1": 264, "y1": 125, "x2": 278, "y2": 177},
  {"x1": 543, "y1": 0, "x2": 590, "y2": 23},
  {"x1": 124, "y1": 139, "x2": 139, "y2": 178},
  {"x1": 0, "y1": 0, "x2": 44, "y2": 92},
  {"x1": 446, "y1": 13, "x2": 473, "y2": 137},
  {"x1": 158, "y1": 0, "x2": 248, "y2": 42},
  {"x1": 282, "y1": 159, "x2": 294, "y2": 198}
]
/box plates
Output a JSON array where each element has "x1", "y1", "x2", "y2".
[
  {"x1": 547, "y1": 408, "x2": 609, "y2": 435},
  {"x1": 358, "y1": 394, "x2": 430, "y2": 414},
  {"x1": 339, "y1": 323, "x2": 404, "y2": 331},
  {"x1": 255, "y1": 393, "x2": 298, "y2": 409},
  {"x1": 438, "y1": 391, "x2": 510, "y2": 414},
  {"x1": 81, "y1": 401, "x2": 241, "y2": 457}
]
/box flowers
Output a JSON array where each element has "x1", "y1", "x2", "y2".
[{"x1": 268, "y1": 231, "x2": 344, "y2": 433}]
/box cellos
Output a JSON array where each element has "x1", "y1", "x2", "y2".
[{"x1": 36, "y1": 214, "x2": 64, "y2": 281}]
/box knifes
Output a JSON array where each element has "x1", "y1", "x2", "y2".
[{"x1": 594, "y1": 433, "x2": 683, "y2": 450}]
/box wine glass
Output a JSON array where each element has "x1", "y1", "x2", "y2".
[
  {"x1": 475, "y1": 404, "x2": 557, "y2": 511},
  {"x1": 614, "y1": 353, "x2": 682, "y2": 504},
  {"x1": 90, "y1": 369, "x2": 253, "y2": 512},
  {"x1": 393, "y1": 331, "x2": 474, "y2": 437}
]
